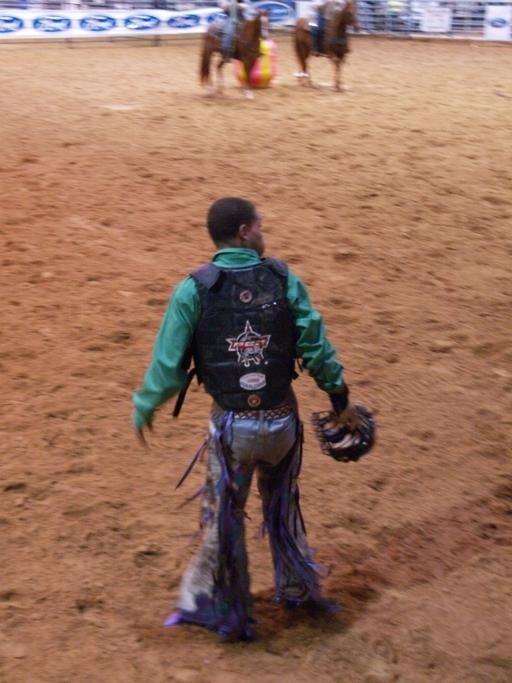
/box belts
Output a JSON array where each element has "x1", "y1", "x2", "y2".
[{"x1": 232, "y1": 404, "x2": 293, "y2": 421}]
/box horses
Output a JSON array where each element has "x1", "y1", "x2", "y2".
[
  {"x1": 198, "y1": 7, "x2": 271, "y2": 100},
  {"x1": 293, "y1": 0, "x2": 360, "y2": 91}
]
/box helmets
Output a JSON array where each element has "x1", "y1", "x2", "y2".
[{"x1": 310, "y1": 404, "x2": 375, "y2": 462}]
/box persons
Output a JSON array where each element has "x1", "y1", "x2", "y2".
[
  {"x1": 221, "y1": 0, "x2": 248, "y2": 58},
  {"x1": 311, "y1": 0, "x2": 348, "y2": 51},
  {"x1": 130, "y1": 198, "x2": 358, "y2": 643}
]
